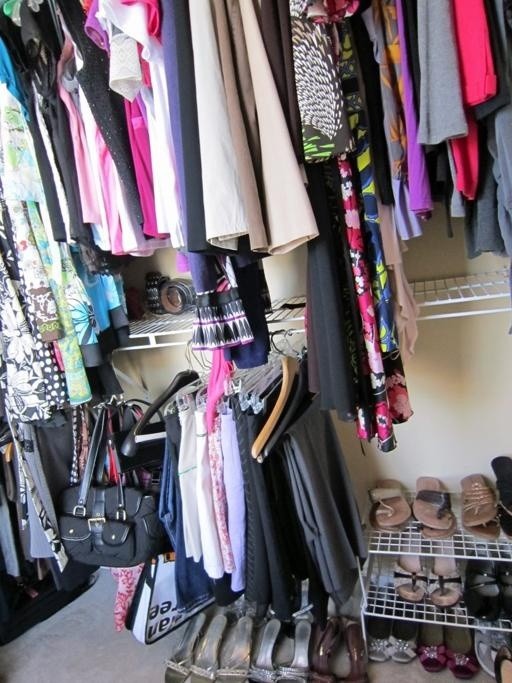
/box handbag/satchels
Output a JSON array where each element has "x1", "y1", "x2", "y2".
[
  {"x1": 125, "y1": 551, "x2": 217, "y2": 645},
  {"x1": 56, "y1": 404, "x2": 165, "y2": 567}
]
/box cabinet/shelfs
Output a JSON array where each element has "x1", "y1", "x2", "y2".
[{"x1": 356, "y1": 489, "x2": 512, "y2": 665}]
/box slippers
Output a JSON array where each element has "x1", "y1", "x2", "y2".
[
  {"x1": 369, "y1": 455, "x2": 511, "y2": 538},
  {"x1": 165, "y1": 603, "x2": 369, "y2": 682}
]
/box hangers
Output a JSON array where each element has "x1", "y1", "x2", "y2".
[{"x1": 119, "y1": 328, "x2": 316, "y2": 464}]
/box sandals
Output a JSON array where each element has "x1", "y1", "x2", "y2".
[{"x1": 367, "y1": 529, "x2": 512, "y2": 682}]
